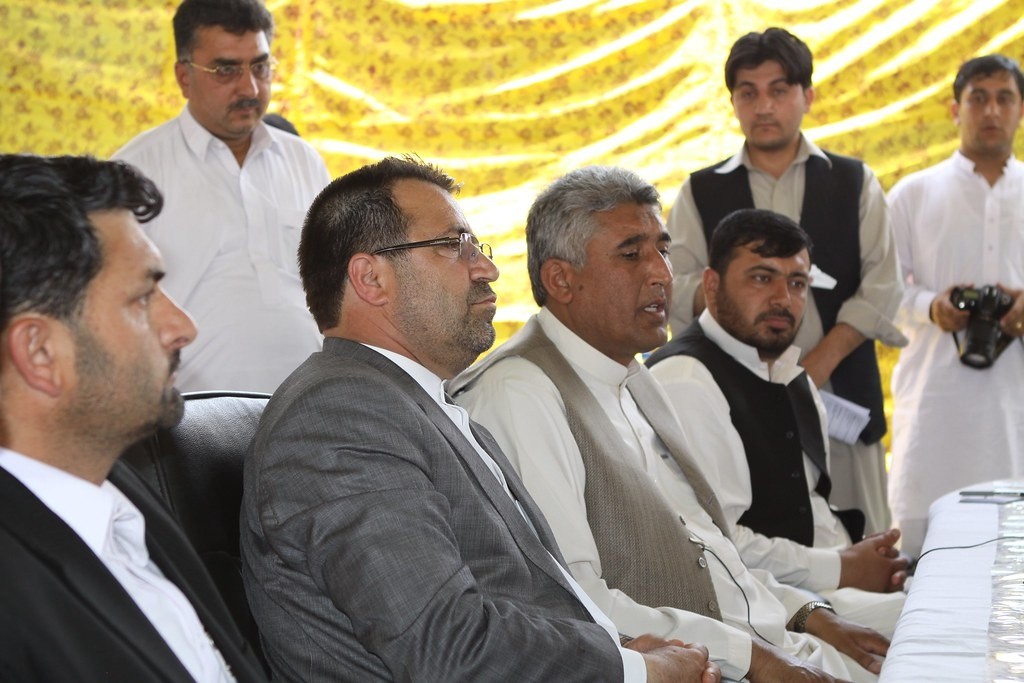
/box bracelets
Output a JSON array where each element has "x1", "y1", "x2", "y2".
[{"x1": 795, "y1": 602, "x2": 837, "y2": 633}]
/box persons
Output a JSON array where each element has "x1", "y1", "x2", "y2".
[
  {"x1": 446, "y1": 166, "x2": 892, "y2": 683},
  {"x1": 885, "y1": 54, "x2": 1024, "y2": 562},
  {"x1": 236, "y1": 159, "x2": 728, "y2": 683},
  {"x1": 108, "y1": 0, "x2": 339, "y2": 395},
  {"x1": 0, "y1": 156, "x2": 276, "y2": 683},
  {"x1": 644, "y1": 210, "x2": 919, "y2": 644},
  {"x1": 670, "y1": 27, "x2": 909, "y2": 543}
]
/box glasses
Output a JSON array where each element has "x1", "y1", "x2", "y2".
[
  {"x1": 183, "y1": 60, "x2": 277, "y2": 85},
  {"x1": 371, "y1": 230, "x2": 492, "y2": 263}
]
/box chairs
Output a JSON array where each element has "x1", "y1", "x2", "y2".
[{"x1": 125, "y1": 388, "x2": 273, "y2": 679}]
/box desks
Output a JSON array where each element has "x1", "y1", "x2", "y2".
[{"x1": 877, "y1": 479, "x2": 1024, "y2": 682}]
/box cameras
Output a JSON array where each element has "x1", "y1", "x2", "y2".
[{"x1": 951, "y1": 285, "x2": 1017, "y2": 368}]
